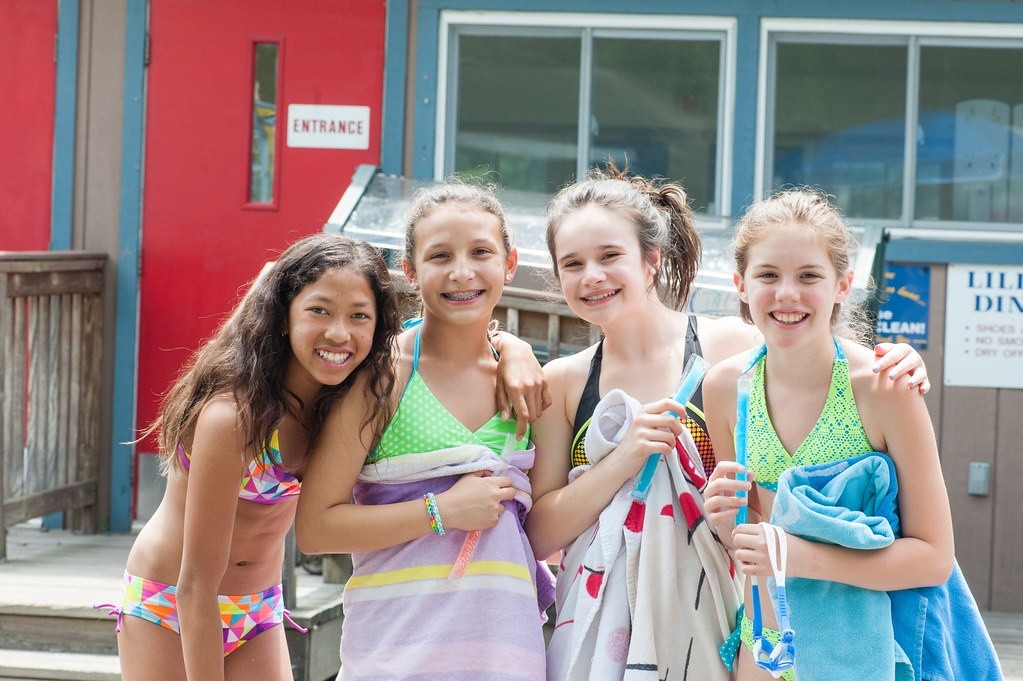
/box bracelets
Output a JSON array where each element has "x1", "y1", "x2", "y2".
[{"x1": 423, "y1": 491, "x2": 445, "y2": 537}]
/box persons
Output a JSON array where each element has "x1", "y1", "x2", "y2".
[
  {"x1": 701, "y1": 183, "x2": 1006, "y2": 680},
  {"x1": 295, "y1": 173, "x2": 558, "y2": 681},
  {"x1": 525, "y1": 157, "x2": 930, "y2": 681},
  {"x1": 94, "y1": 234, "x2": 553, "y2": 681}
]
